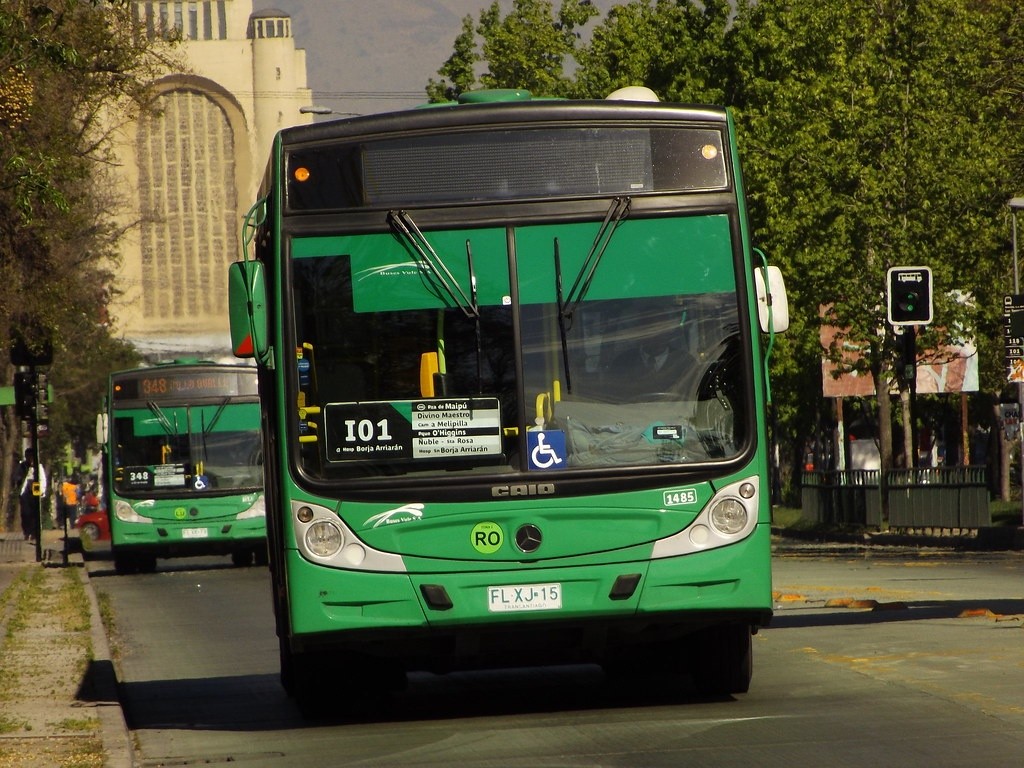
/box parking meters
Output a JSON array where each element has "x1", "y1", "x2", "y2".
[{"x1": 26, "y1": 479, "x2": 40, "y2": 499}]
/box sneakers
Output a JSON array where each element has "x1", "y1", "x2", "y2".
[{"x1": 24, "y1": 534, "x2": 40, "y2": 546}]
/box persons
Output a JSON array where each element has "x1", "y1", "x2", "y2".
[
  {"x1": 929, "y1": 382, "x2": 1024, "y2": 501},
  {"x1": 609, "y1": 306, "x2": 699, "y2": 404},
  {"x1": 49, "y1": 469, "x2": 99, "y2": 529},
  {"x1": 15, "y1": 448, "x2": 46, "y2": 545}
]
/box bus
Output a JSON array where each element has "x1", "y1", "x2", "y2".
[
  {"x1": 226, "y1": 82, "x2": 790, "y2": 708},
  {"x1": 94, "y1": 356, "x2": 272, "y2": 575}
]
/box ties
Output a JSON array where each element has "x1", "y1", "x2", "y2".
[{"x1": 646, "y1": 358, "x2": 656, "y2": 393}]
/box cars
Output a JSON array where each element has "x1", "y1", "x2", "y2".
[{"x1": 72, "y1": 497, "x2": 111, "y2": 542}]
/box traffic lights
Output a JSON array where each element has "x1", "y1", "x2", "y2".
[{"x1": 886, "y1": 265, "x2": 933, "y2": 325}]
